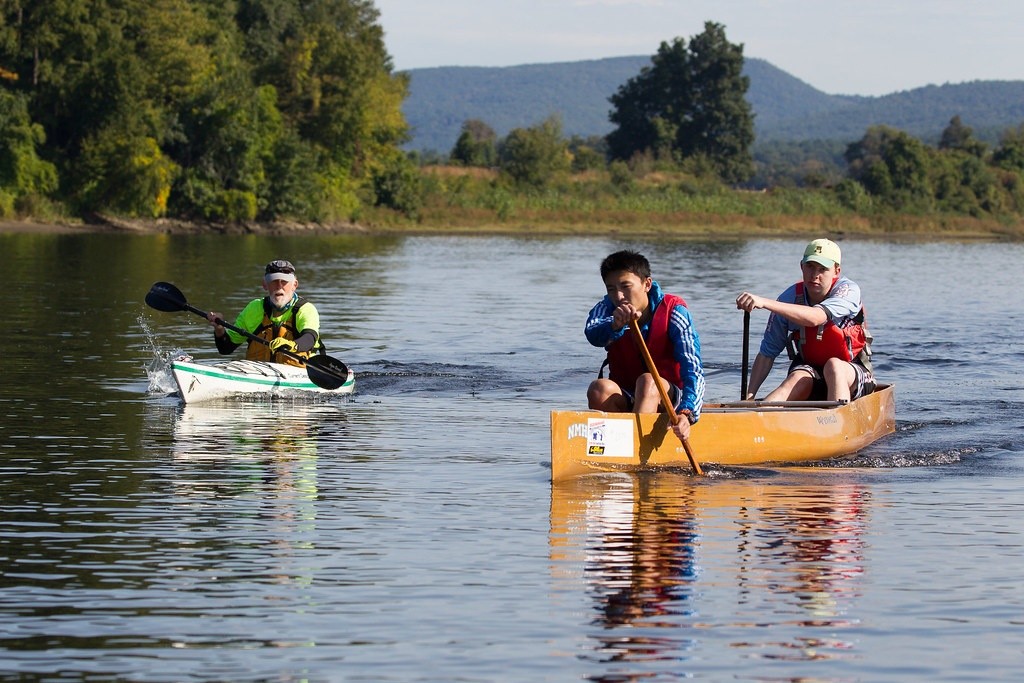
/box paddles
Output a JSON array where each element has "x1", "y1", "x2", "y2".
[
  {"x1": 702, "y1": 399, "x2": 848, "y2": 409},
  {"x1": 143, "y1": 279, "x2": 351, "y2": 392},
  {"x1": 740, "y1": 306, "x2": 751, "y2": 402},
  {"x1": 627, "y1": 317, "x2": 705, "y2": 476}
]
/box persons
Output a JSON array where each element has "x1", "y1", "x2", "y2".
[
  {"x1": 585, "y1": 249, "x2": 705, "y2": 440},
  {"x1": 207, "y1": 260, "x2": 320, "y2": 368},
  {"x1": 736, "y1": 239, "x2": 877, "y2": 408}
]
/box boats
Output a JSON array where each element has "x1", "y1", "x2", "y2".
[
  {"x1": 169, "y1": 360, "x2": 353, "y2": 402},
  {"x1": 547, "y1": 380, "x2": 898, "y2": 483}
]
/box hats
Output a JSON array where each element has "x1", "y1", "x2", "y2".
[
  {"x1": 264, "y1": 260, "x2": 295, "y2": 283},
  {"x1": 801, "y1": 237, "x2": 841, "y2": 268}
]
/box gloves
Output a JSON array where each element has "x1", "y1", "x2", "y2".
[{"x1": 269, "y1": 337, "x2": 297, "y2": 354}]
